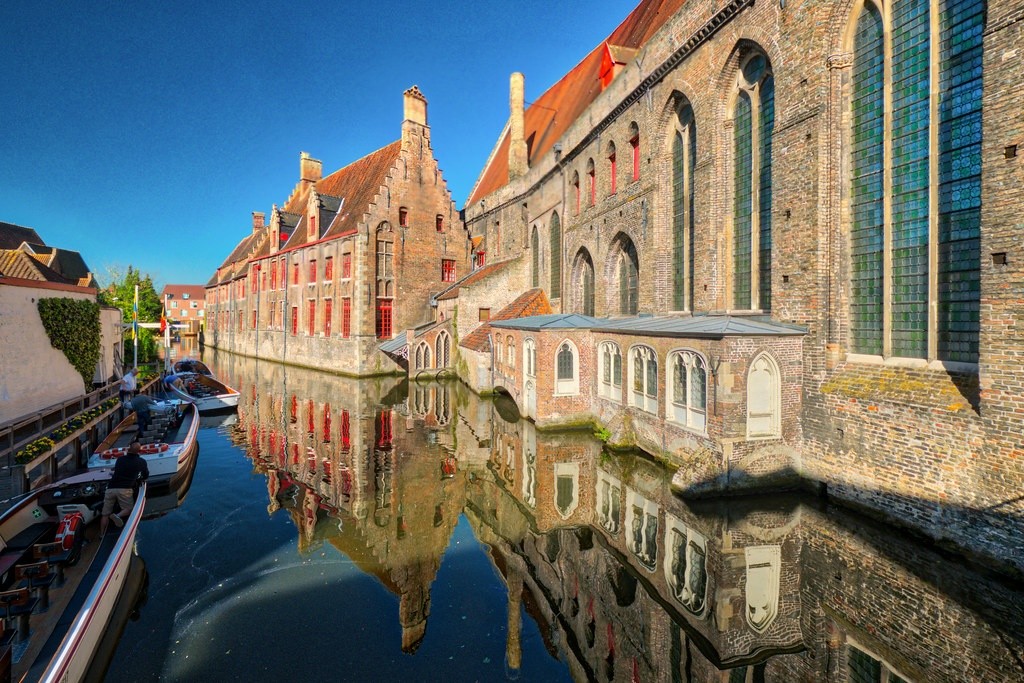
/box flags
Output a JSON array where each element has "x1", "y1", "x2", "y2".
[
  {"x1": 131, "y1": 295, "x2": 138, "y2": 339},
  {"x1": 160, "y1": 302, "x2": 166, "y2": 332}
]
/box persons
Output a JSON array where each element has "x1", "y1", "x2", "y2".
[
  {"x1": 98, "y1": 443, "x2": 149, "y2": 540},
  {"x1": 132, "y1": 389, "x2": 156, "y2": 436},
  {"x1": 162, "y1": 375, "x2": 188, "y2": 393},
  {"x1": 119, "y1": 369, "x2": 138, "y2": 404}
]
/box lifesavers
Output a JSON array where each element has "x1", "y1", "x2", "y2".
[
  {"x1": 99, "y1": 446, "x2": 130, "y2": 459},
  {"x1": 55, "y1": 511, "x2": 82, "y2": 548},
  {"x1": 139, "y1": 443, "x2": 169, "y2": 454}
]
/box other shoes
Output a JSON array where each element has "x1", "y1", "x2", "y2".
[{"x1": 110, "y1": 513, "x2": 123, "y2": 527}]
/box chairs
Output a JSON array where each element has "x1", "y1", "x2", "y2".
[
  {"x1": 187, "y1": 378, "x2": 218, "y2": 396},
  {"x1": 138, "y1": 417, "x2": 170, "y2": 444},
  {"x1": 0, "y1": 540, "x2": 73, "y2": 646}
]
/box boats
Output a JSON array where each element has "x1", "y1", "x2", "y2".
[
  {"x1": 168, "y1": 359, "x2": 241, "y2": 412},
  {"x1": 0, "y1": 468, "x2": 147, "y2": 683},
  {"x1": 86, "y1": 398, "x2": 200, "y2": 477}
]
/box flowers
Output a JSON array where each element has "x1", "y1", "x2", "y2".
[{"x1": 14, "y1": 396, "x2": 119, "y2": 463}]
